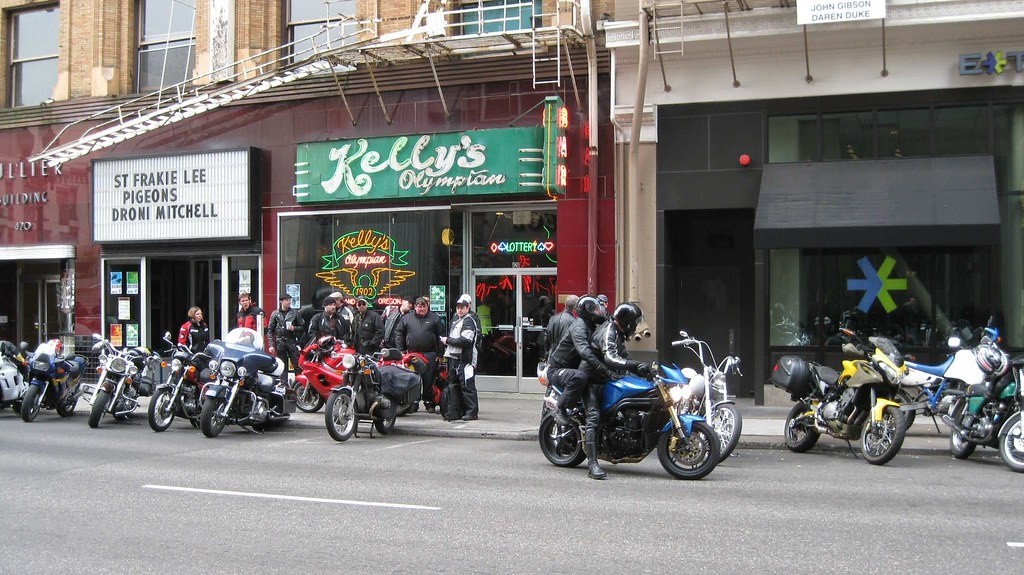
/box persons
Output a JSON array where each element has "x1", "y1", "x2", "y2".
[
  {"x1": 476, "y1": 296, "x2": 556, "y2": 362},
  {"x1": 296, "y1": 290, "x2": 481, "y2": 420},
  {"x1": 178, "y1": 307, "x2": 210, "y2": 354},
  {"x1": 545, "y1": 294, "x2": 648, "y2": 478},
  {"x1": 237, "y1": 293, "x2": 268, "y2": 336},
  {"x1": 268, "y1": 293, "x2": 305, "y2": 387}
]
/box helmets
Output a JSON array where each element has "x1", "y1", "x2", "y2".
[
  {"x1": 976, "y1": 346, "x2": 1008, "y2": 374},
  {"x1": 575, "y1": 293, "x2": 606, "y2": 324},
  {"x1": 48, "y1": 339, "x2": 63, "y2": 357},
  {"x1": 537, "y1": 362, "x2": 551, "y2": 385},
  {"x1": 613, "y1": 303, "x2": 642, "y2": 332}
]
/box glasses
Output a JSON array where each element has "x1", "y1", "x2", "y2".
[{"x1": 358, "y1": 303, "x2": 367, "y2": 306}]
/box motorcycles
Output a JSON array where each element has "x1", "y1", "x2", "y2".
[
  {"x1": 949, "y1": 353, "x2": 1024, "y2": 474},
  {"x1": 289, "y1": 334, "x2": 429, "y2": 413},
  {"x1": 79, "y1": 333, "x2": 157, "y2": 429},
  {"x1": 769, "y1": 326, "x2": 909, "y2": 467},
  {"x1": 148, "y1": 331, "x2": 202, "y2": 433},
  {"x1": 670, "y1": 330, "x2": 744, "y2": 464},
  {"x1": 19, "y1": 339, "x2": 90, "y2": 423},
  {"x1": 0, "y1": 340, "x2": 29, "y2": 415},
  {"x1": 538, "y1": 362, "x2": 722, "y2": 482},
  {"x1": 896, "y1": 315, "x2": 1010, "y2": 437},
  {"x1": 190, "y1": 327, "x2": 298, "y2": 438},
  {"x1": 324, "y1": 348, "x2": 424, "y2": 442}
]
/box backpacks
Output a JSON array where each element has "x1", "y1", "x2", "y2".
[{"x1": 441, "y1": 382, "x2": 466, "y2": 421}]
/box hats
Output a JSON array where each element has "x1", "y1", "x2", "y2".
[
  {"x1": 330, "y1": 292, "x2": 343, "y2": 299},
  {"x1": 456, "y1": 299, "x2": 469, "y2": 307},
  {"x1": 279, "y1": 293, "x2": 292, "y2": 300},
  {"x1": 357, "y1": 296, "x2": 365, "y2": 301},
  {"x1": 464, "y1": 293, "x2": 472, "y2": 304},
  {"x1": 596, "y1": 294, "x2": 607, "y2": 305},
  {"x1": 322, "y1": 296, "x2": 336, "y2": 306}
]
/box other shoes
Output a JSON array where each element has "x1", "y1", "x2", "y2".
[
  {"x1": 409, "y1": 406, "x2": 417, "y2": 412},
  {"x1": 429, "y1": 406, "x2": 435, "y2": 413},
  {"x1": 463, "y1": 412, "x2": 476, "y2": 420}
]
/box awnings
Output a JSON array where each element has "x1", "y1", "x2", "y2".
[{"x1": 753, "y1": 155, "x2": 1003, "y2": 243}]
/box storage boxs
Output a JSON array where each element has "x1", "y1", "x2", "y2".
[
  {"x1": 138, "y1": 375, "x2": 156, "y2": 396},
  {"x1": 769, "y1": 354, "x2": 806, "y2": 393},
  {"x1": 273, "y1": 387, "x2": 297, "y2": 414},
  {"x1": 394, "y1": 372, "x2": 422, "y2": 405}
]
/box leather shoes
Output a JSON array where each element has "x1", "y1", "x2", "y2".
[{"x1": 554, "y1": 407, "x2": 569, "y2": 426}]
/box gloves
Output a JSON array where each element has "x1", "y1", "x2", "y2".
[
  {"x1": 604, "y1": 369, "x2": 624, "y2": 382},
  {"x1": 635, "y1": 362, "x2": 650, "y2": 375}
]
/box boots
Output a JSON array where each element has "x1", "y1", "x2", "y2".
[{"x1": 585, "y1": 427, "x2": 607, "y2": 479}]
